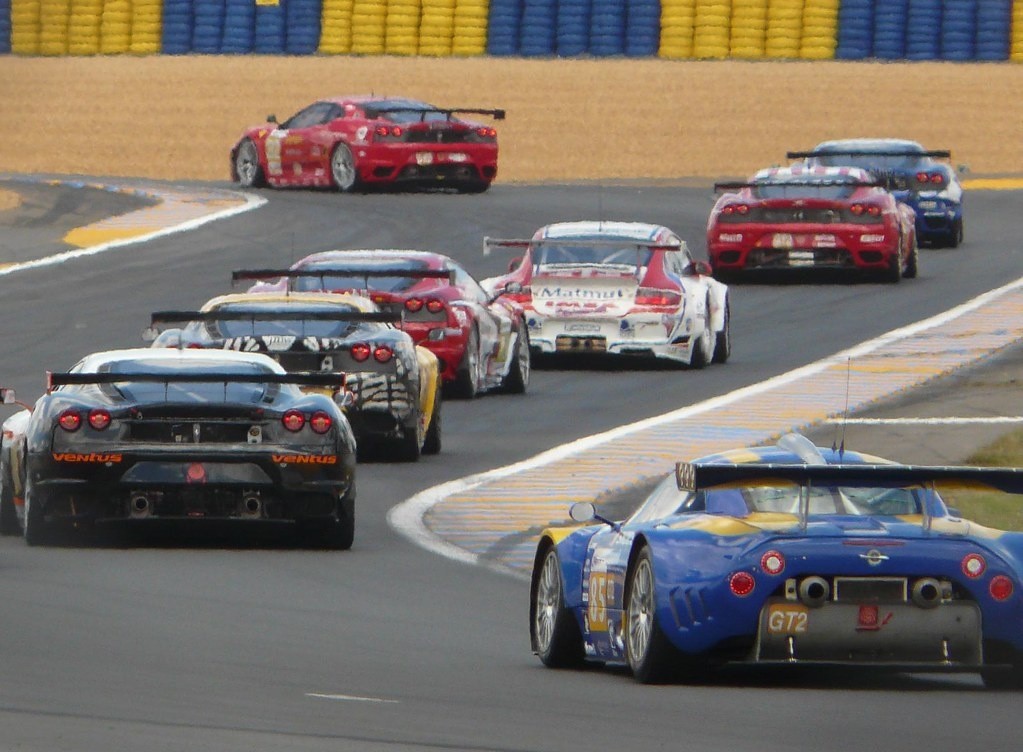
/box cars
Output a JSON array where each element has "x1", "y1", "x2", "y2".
[
  {"x1": 531, "y1": 434, "x2": 1022, "y2": 688},
  {"x1": 0, "y1": 346, "x2": 359, "y2": 554},
  {"x1": 150, "y1": 291, "x2": 442, "y2": 462},
  {"x1": 230, "y1": 246, "x2": 532, "y2": 400},
  {"x1": 479, "y1": 219, "x2": 732, "y2": 369},
  {"x1": 228, "y1": 99, "x2": 508, "y2": 195},
  {"x1": 788, "y1": 138, "x2": 971, "y2": 250},
  {"x1": 705, "y1": 164, "x2": 920, "y2": 286}
]
